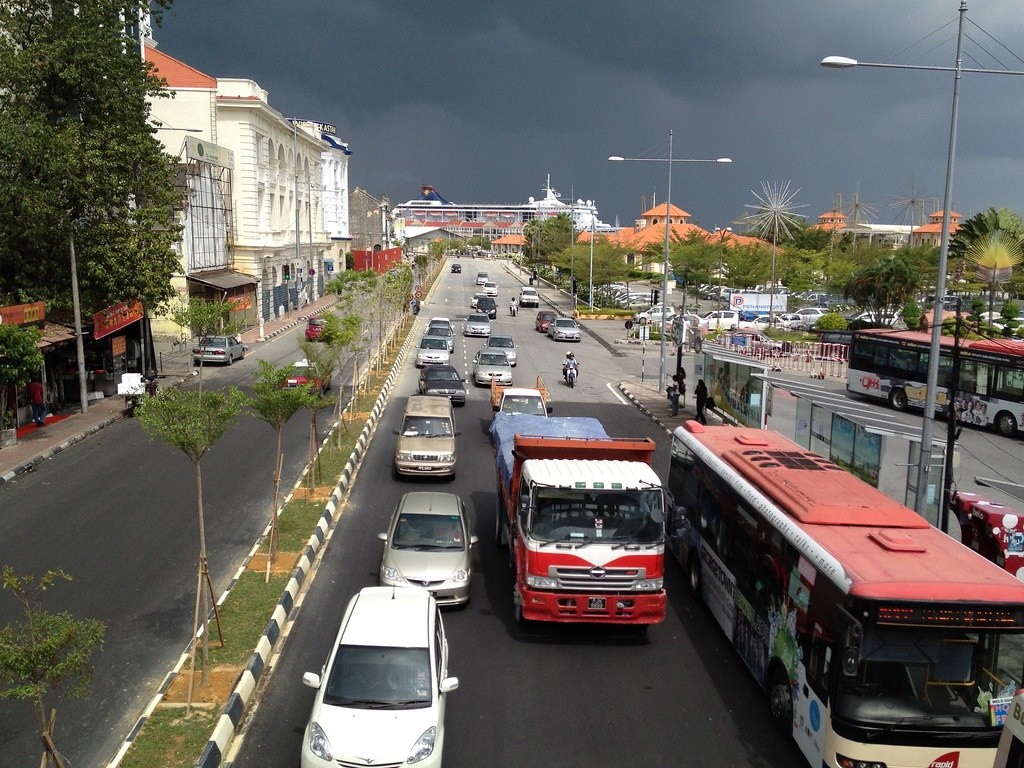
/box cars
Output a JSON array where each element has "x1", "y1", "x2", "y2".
[
  {"x1": 697, "y1": 283, "x2": 857, "y2": 307},
  {"x1": 377, "y1": 492, "x2": 479, "y2": 607},
  {"x1": 510, "y1": 287, "x2": 581, "y2": 343},
  {"x1": 451, "y1": 264, "x2": 500, "y2": 337},
  {"x1": 391, "y1": 317, "x2": 554, "y2": 417},
  {"x1": 845, "y1": 311, "x2": 902, "y2": 326},
  {"x1": 701, "y1": 308, "x2": 830, "y2": 357},
  {"x1": 190, "y1": 335, "x2": 245, "y2": 366},
  {"x1": 281, "y1": 315, "x2": 338, "y2": 395},
  {"x1": 301, "y1": 586, "x2": 458, "y2": 768},
  {"x1": 592, "y1": 282, "x2": 707, "y2": 332}
]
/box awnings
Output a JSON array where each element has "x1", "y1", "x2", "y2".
[{"x1": 187, "y1": 269, "x2": 260, "y2": 292}]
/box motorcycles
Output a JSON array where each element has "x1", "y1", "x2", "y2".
[{"x1": 563, "y1": 361, "x2": 581, "y2": 388}]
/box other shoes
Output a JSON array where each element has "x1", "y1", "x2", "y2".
[
  {"x1": 32, "y1": 417, "x2": 40, "y2": 423},
  {"x1": 667, "y1": 405, "x2": 673, "y2": 411},
  {"x1": 35, "y1": 420, "x2": 46, "y2": 427}
]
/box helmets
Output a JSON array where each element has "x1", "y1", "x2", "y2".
[
  {"x1": 566, "y1": 351, "x2": 574, "y2": 360},
  {"x1": 512, "y1": 297, "x2": 515, "y2": 301}
]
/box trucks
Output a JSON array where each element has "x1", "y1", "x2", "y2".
[{"x1": 493, "y1": 412, "x2": 688, "y2": 624}]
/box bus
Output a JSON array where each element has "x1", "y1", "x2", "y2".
[
  {"x1": 665, "y1": 420, "x2": 1024, "y2": 768},
  {"x1": 846, "y1": 328, "x2": 1024, "y2": 439}
]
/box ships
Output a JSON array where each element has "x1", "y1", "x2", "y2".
[{"x1": 391, "y1": 185, "x2": 613, "y2": 240}]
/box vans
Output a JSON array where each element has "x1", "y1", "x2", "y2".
[{"x1": 393, "y1": 396, "x2": 460, "y2": 480}]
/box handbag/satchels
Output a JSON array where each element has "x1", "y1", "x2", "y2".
[{"x1": 705, "y1": 397, "x2": 716, "y2": 408}]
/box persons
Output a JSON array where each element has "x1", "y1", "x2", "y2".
[
  {"x1": 668, "y1": 374, "x2": 680, "y2": 418},
  {"x1": 947, "y1": 399, "x2": 988, "y2": 426},
  {"x1": 510, "y1": 297, "x2": 518, "y2": 315},
  {"x1": 695, "y1": 379, "x2": 707, "y2": 425},
  {"x1": 530, "y1": 269, "x2": 534, "y2": 278},
  {"x1": 563, "y1": 351, "x2": 578, "y2": 380},
  {"x1": 27, "y1": 376, "x2": 46, "y2": 427},
  {"x1": 740, "y1": 380, "x2": 754, "y2": 414}
]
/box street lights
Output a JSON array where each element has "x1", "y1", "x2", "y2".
[
  {"x1": 608, "y1": 156, "x2": 746, "y2": 394},
  {"x1": 714, "y1": 227, "x2": 733, "y2": 328},
  {"x1": 821, "y1": 56, "x2": 1023, "y2": 521}
]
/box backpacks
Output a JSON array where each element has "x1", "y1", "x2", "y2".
[{"x1": 676, "y1": 381, "x2": 686, "y2": 394}]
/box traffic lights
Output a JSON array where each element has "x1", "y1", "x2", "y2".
[{"x1": 284, "y1": 266, "x2": 290, "y2": 279}]
